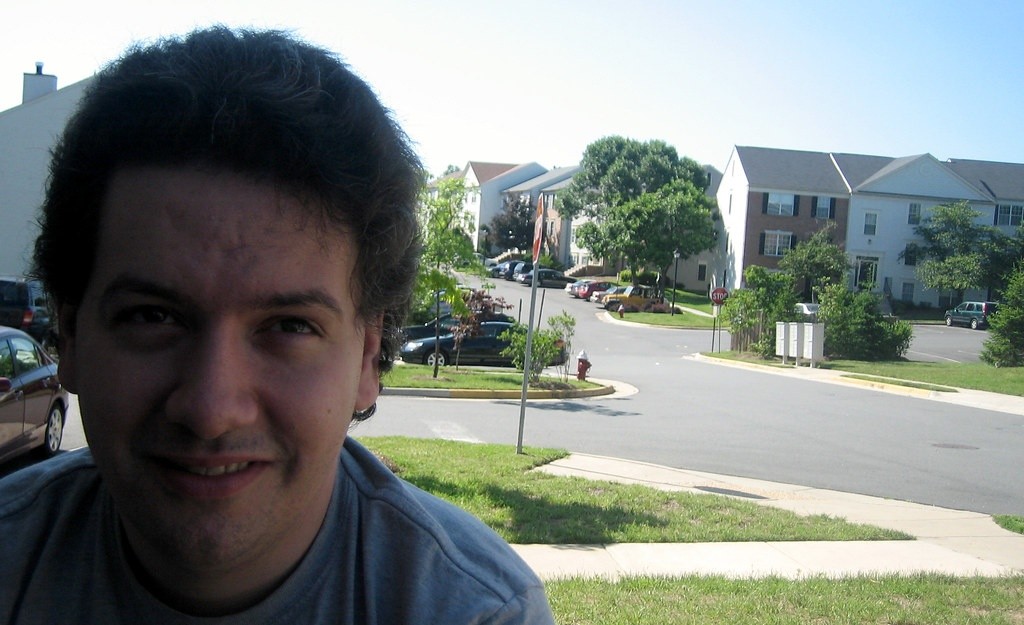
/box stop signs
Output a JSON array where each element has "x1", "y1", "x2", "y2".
[
  {"x1": 710, "y1": 288, "x2": 729, "y2": 305},
  {"x1": 532, "y1": 191, "x2": 546, "y2": 264}
]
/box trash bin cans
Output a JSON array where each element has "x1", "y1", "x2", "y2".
[
  {"x1": 775, "y1": 321, "x2": 790, "y2": 364},
  {"x1": 788, "y1": 322, "x2": 804, "y2": 365},
  {"x1": 802, "y1": 322, "x2": 825, "y2": 369}
]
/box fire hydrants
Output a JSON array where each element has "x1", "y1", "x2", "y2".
[
  {"x1": 575, "y1": 350, "x2": 592, "y2": 382},
  {"x1": 618, "y1": 304, "x2": 626, "y2": 319}
]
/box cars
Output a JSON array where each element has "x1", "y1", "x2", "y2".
[
  {"x1": 396, "y1": 322, "x2": 566, "y2": 369},
  {"x1": 566, "y1": 279, "x2": 669, "y2": 312},
  {"x1": 449, "y1": 253, "x2": 498, "y2": 267},
  {"x1": 486, "y1": 253, "x2": 577, "y2": 288},
  {"x1": 795, "y1": 303, "x2": 821, "y2": 318},
  {"x1": 944, "y1": 302, "x2": 998, "y2": 330},
  {"x1": 0, "y1": 278, "x2": 51, "y2": 340},
  {"x1": 1, "y1": 330, "x2": 67, "y2": 476},
  {"x1": 399, "y1": 288, "x2": 516, "y2": 344}
]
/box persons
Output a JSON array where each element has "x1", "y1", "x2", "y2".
[{"x1": 0, "y1": 26, "x2": 554, "y2": 625}]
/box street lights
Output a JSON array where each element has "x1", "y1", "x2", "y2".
[{"x1": 672, "y1": 248, "x2": 681, "y2": 316}]
